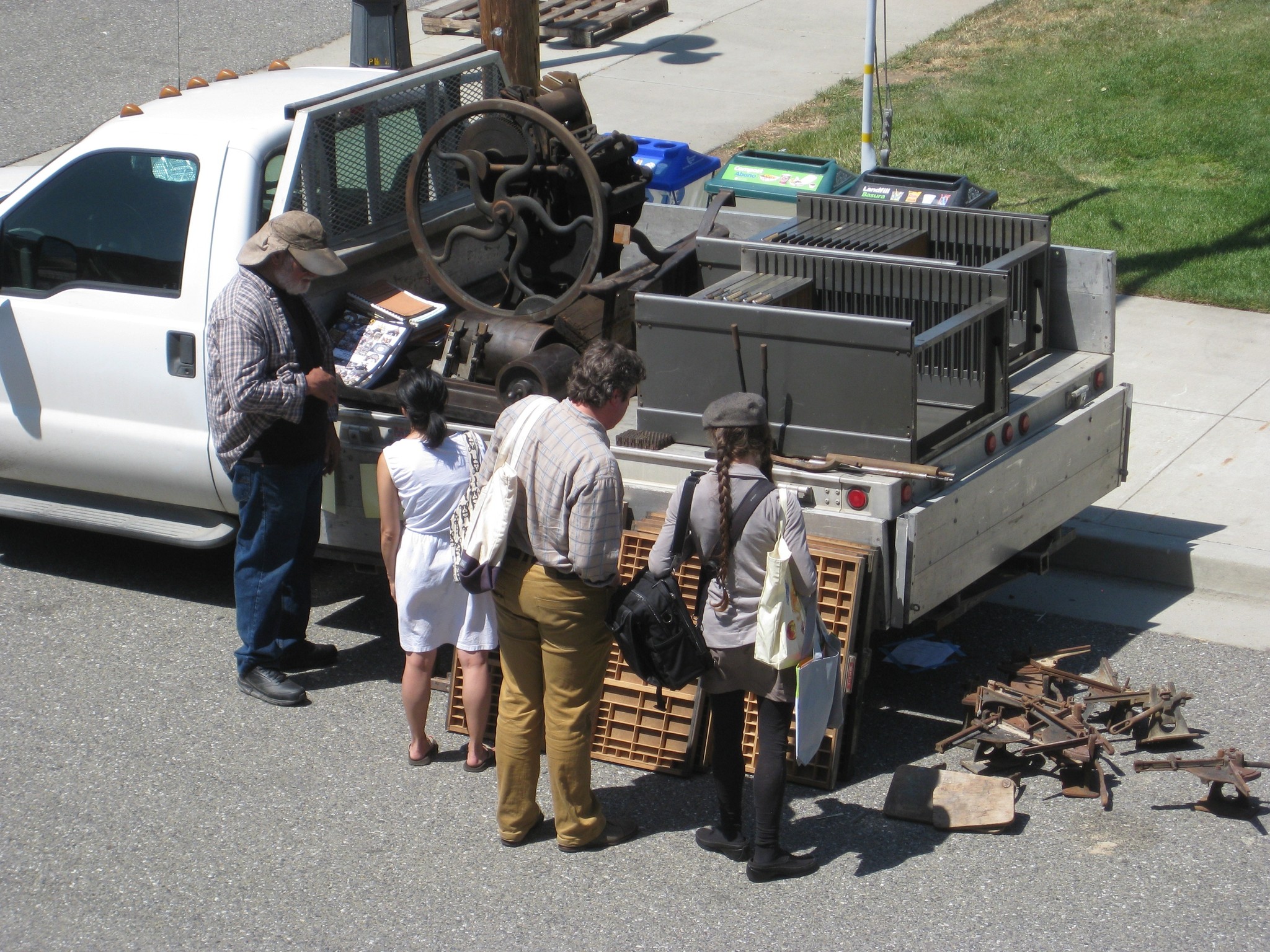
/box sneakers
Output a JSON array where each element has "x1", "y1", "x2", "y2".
[
  {"x1": 237, "y1": 663, "x2": 306, "y2": 706},
  {"x1": 277, "y1": 640, "x2": 338, "y2": 672},
  {"x1": 500, "y1": 811, "x2": 544, "y2": 847},
  {"x1": 558, "y1": 816, "x2": 638, "y2": 853}
]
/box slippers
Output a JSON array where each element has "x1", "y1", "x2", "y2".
[
  {"x1": 408, "y1": 737, "x2": 438, "y2": 766},
  {"x1": 463, "y1": 743, "x2": 495, "y2": 772}
]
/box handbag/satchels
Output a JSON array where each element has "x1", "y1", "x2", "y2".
[
  {"x1": 796, "y1": 560, "x2": 843, "y2": 767},
  {"x1": 753, "y1": 488, "x2": 807, "y2": 670}
]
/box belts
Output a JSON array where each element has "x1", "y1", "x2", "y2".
[{"x1": 506, "y1": 546, "x2": 543, "y2": 566}]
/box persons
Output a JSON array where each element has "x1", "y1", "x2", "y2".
[
  {"x1": 475, "y1": 340, "x2": 649, "y2": 857},
  {"x1": 205, "y1": 211, "x2": 343, "y2": 706},
  {"x1": 645, "y1": 391, "x2": 820, "y2": 884},
  {"x1": 376, "y1": 367, "x2": 500, "y2": 772}
]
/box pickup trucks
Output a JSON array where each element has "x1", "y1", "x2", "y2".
[{"x1": 0, "y1": 63, "x2": 1131, "y2": 656}]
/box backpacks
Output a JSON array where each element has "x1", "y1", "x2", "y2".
[
  {"x1": 603, "y1": 470, "x2": 778, "y2": 692},
  {"x1": 449, "y1": 396, "x2": 560, "y2": 594}
]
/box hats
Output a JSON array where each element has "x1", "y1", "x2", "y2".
[
  {"x1": 236, "y1": 210, "x2": 348, "y2": 276},
  {"x1": 701, "y1": 391, "x2": 768, "y2": 431}
]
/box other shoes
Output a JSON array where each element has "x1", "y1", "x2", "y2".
[
  {"x1": 746, "y1": 852, "x2": 818, "y2": 883},
  {"x1": 695, "y1": 826, "x2": 750, "y2": 861}
]
